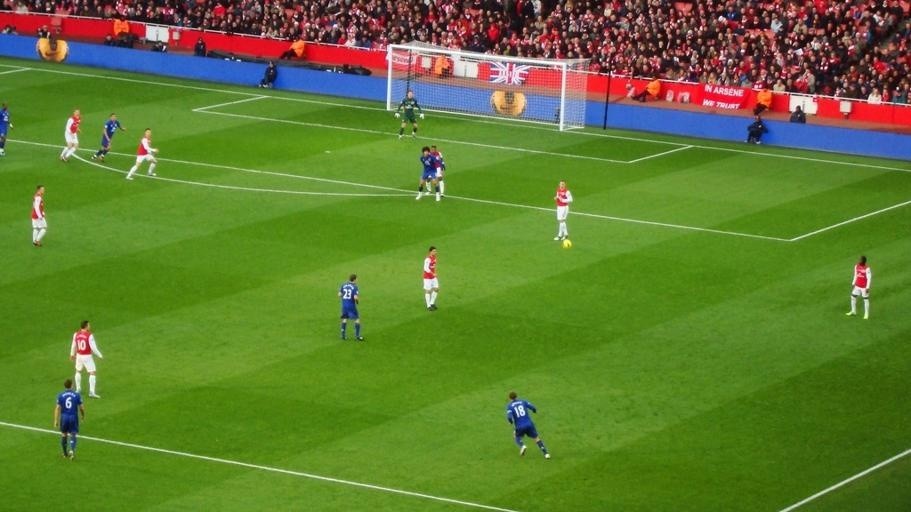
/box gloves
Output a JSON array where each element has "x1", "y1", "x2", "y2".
[
  {"x1": 419, "y1": 114, "x2": 424, "y2": 119},
  {"x1": 394, "y1": 112, "x2": 399, "y2": 118}
]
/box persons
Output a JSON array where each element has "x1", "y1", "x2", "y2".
[
  {"x1": 29, "y1": 184, "x2": 49, "y2": 249},
  {"x1": 745, "y1": 112, "x2": 771, "y2": 149},
  {"x1": 751, "y1": 84, "x2": 773, "y2": 116},
  {"x1": 844, "y1": 252, "x2": 874, "y2": 323},
  {"x1": 396, "y1": 87, "x2": 426, "y2": 138},
  {"x1": 58, "y1": 108, "x2": 85, "y2": 163},
  {"x1": 52, "y1": 378, "x2": 87, "y2": 461},
  {"x1": 788, "y1": 102, "x2": 811, "y2": 129},
  {"x1": 2, "y1": 1, "x2": 911, "y2": 105},
  {"x1": 67, "y1": 320, "x2": 106, "y2": 402},
  {"x1": 421, "y1": 244, "x2": 445, "y2": 313},
  {"x1": 1, "y1": 102, "x2": 15, "y2": 157},
  {"x1": 550, "y1": 175, "x2": 575, "y2": 245},
  {"x1": 89, "y1": 112, "x2": 127, "y2": 161},
  {"x1": 506, "y1": 390, "x2": 554, "y2": 462},
  {"x1": 124, "y1": 129, "x2": 162, "y2": 184},
  {"x1": 336, "y1": 273, "x2": 368, "y2": 344}
]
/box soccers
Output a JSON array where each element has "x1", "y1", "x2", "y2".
[
  {"x1": 491, "y1": 90, "x2": 526, "y2": 117},
  {"x1": 35, "y1": 38, "x2": 68, "y2": 62},
  {"x1": 563, "y1": 239, "x2": 571, "y2": 247}
]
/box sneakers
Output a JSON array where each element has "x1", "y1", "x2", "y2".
[
  {"x1": 67, "y1": 394, "x2": 99, "y2": 462},
  {"x1": 415, "y1": 190, "x2": 444, "y2": 202},
  {"x1": 0, "y1": 149, "x2": 159, "y2": 247},
  {"x1": 845, "y1": 311, "x2": 870, "y2": 320},
  {"x1": 426, "y1": 303, "x2": 437, "y2": 311},
  {"x1": 554, "y1": 235, "x2": 568, "y2": 241},
  {"x1": 520, "y1": 446, "x2": 551, "y2": 459}
]
